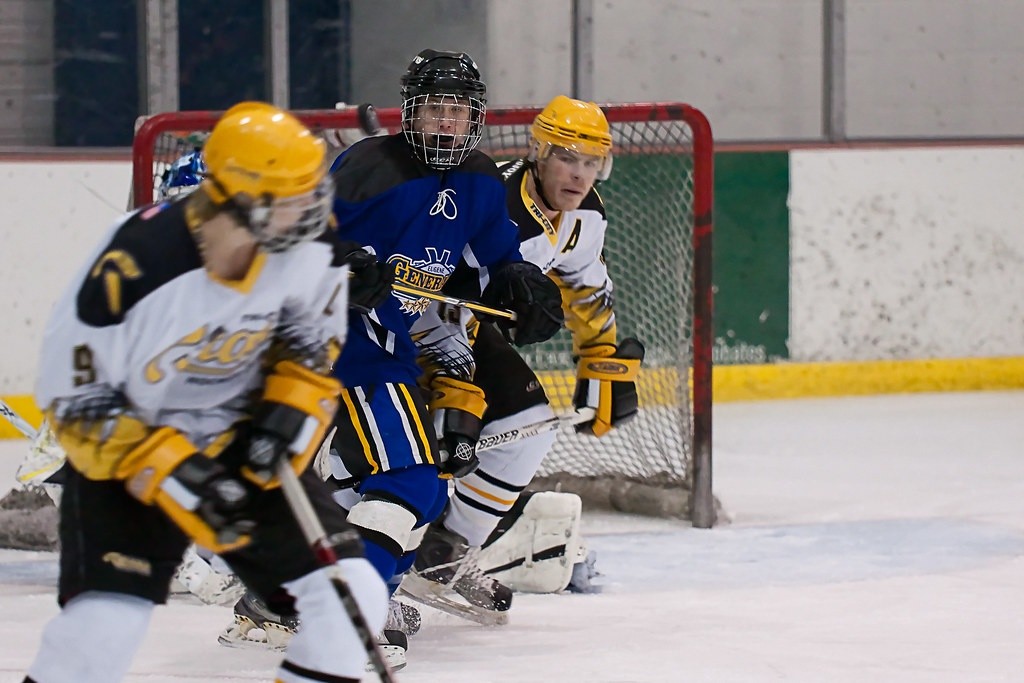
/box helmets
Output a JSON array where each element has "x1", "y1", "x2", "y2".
[
  {"x1": 528, "y1": 95, "x2": 613, "y2": 180},
  {"x1": 399, "y1": 48, "x2": 488, "y2": 169},
  {"x1": 202, "y1": 101, "x2": 329, "y2": 253}
]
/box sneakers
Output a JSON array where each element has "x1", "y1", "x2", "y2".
[
  {"x1": 217, "y1": 589, "x2": 301, "y2": 652},
  {"x1": 400, "y1": 495, "x2": 513, "y2": 625},
  {"x1": 363, "y1": 630, "x2": 408, "y2": 672}
]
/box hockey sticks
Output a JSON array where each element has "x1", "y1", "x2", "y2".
[
  {"x1": 388, "y1": 281, "x2": 515, "y2": 322},
  {"x1": 318, "y1": 409, "x2": 595, "y2": 495},
  {"x1": 277, "y1": 455, "x2": 396, "y2": 683},
  {"x1": 0, "y1": 400, "x2": 38, "y2": 440}
]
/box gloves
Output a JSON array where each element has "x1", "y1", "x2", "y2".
[
  {"x1": 573, "y1": 337, "x2": 645, "y2": 438},
  {"x1": 114, "y1": 426, "x2": 255, "y2": 553},
  {"x1": 335, "y1": 241, "x2": 393, "y2": 307},
  {"x1": 497, "y1": 261, "x2": 564, "y2": 347},
  {"x1": 425, "y1": 374, "x2": 489, "y2": 480},
  {"x1": 240, "y1": 360, "x2": 342, "y2": 490}
]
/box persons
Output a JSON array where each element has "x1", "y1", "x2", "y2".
[
  {"x1": 218, "y1": 50, "x2": 564, "y2": 669},
  {"x1": 21, "y1": 101, "x2": 388, "y2": 683},
  {"x1": 386, "y1": 93, "x2": 645, "y2": 636}
]
